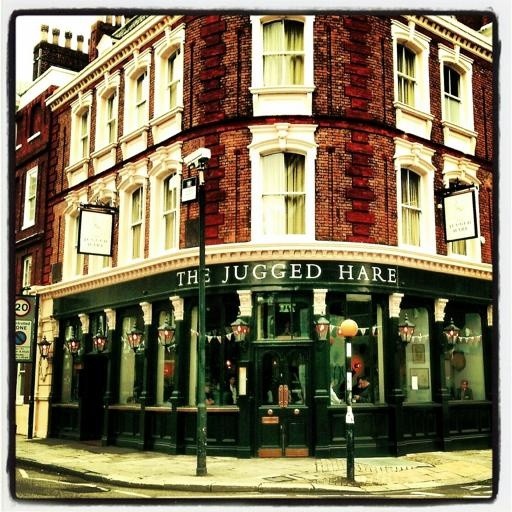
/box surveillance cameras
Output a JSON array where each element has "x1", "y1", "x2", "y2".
[{"x1": 183, "y1": 147, "x2": 212, "y2": 166}]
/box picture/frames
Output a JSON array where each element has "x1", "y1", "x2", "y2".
[
  {"x1": 409, "y1": 368, "x2": 430, "y2": 391},
  {"x1": 411, "y1": 343, "x2": 427, "y2": 366}
]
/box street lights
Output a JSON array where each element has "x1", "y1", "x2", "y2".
[{"x1": 340, "y1": 319, "x2": 360, "y2": 481}]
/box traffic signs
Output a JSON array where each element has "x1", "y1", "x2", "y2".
[{"x1": 15, "y1": 298, "x2": 31, "y2": 316}]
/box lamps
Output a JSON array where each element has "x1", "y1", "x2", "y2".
[
  {"x1": 313, "y1": 311, "x2": 331, "y2": 343},
  {"x1": 155, "y1": 316, "x2": 176, "y2": 348},
  {"x1": 397, "y1": 311, "x2": 415, "y2": 344},
  {"x1": 92, "y1": 324, "x2": 111, "y2": 354},
  {"x1": 441, "y1": 316, "x2": 461, "y2": 348},
  {"x1": 124, "y1": 322, "x2": 146, "y2": 353},
  {"x1": 37, "y1": 335, "x2": 52, "y2": 361},
  {"x1": 230, "y1": 316, "x2": 251, "y2": 344},
  {"x1": 64, "y1": 332, "x2": 81, "y2": 356}
]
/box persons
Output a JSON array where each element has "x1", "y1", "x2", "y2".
[
  {"x1": 224, "y1": 376, "x2": 239, "y2": 405},
  {"x1": 351, "y1": 376, "x2": 374, "y2": 403},
  {"x1": 329, "y1": 376, "x2": 344, "y2": 405},
  {"x1": 205, "y1": 382, "x2": 215, "y2": 405},
  {"x1": 454, "y1": 380, "x2": 473, "y2": 400}
]
